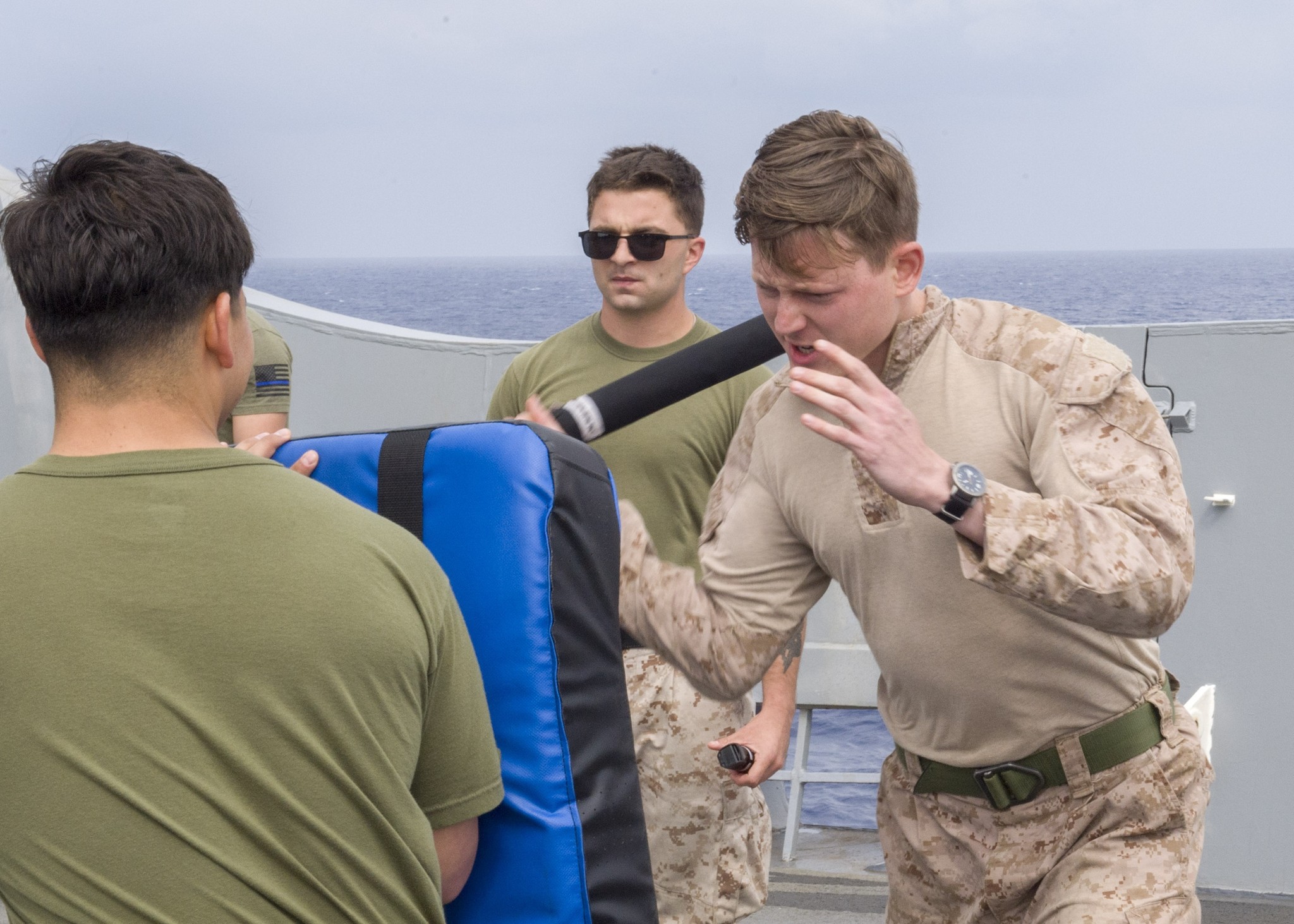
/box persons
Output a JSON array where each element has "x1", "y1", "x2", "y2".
[
  {"x1": 1, "y1": 140, "x2": 504, "y2": 924},
  {"x1": 486, "y1": 146, "x2": 808, "y2": 924},
  {"x1": 514, "y1": 109, "x2": 1207, "y2": 924}
]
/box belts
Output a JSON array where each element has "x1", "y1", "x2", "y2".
[
  {"x1": 896, "y1": 672, "x2": 1174, "y2": 812},
  {"x1": 617, "y1": 626, "x2": 646, "y2": 650}
]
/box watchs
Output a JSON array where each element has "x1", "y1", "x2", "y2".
[{"x1": 930, "y1": 462, "x2": 986, "y2": 525}]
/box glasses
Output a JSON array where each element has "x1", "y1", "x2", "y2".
[{"x1": 578, "y1": 230, "x2": 699, "y2": 261}]
